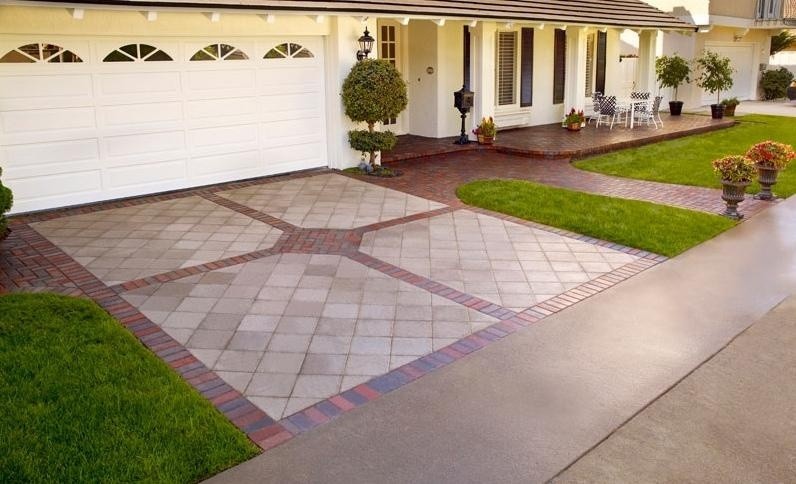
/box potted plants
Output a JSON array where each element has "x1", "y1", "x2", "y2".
[{"x1": 654, "y1": 50, "x2": 740, "y2": 119}]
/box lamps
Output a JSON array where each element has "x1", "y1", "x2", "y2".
[{"x1": 357, "y1": 26, "x2": 375, "y2": 60}]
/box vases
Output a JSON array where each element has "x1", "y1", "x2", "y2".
[
  {"x1": 477, "y1": 134, "x2": 494, "y2": 145},
  {"x1": 719, "y1": 179, "x2": 752, "y2": 220},
  {"x1": 568, "y1": 122, "x2": 581, "y2": 131},
  {"x1": 753, "y1": 167, "x2": 782, "y2": 201}
]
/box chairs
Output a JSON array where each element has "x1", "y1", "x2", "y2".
[{"x1": 588, "y1": 91, "x2": 663, "y2": 131}]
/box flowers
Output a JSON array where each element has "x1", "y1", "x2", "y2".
[
  {"x1": 713, "y1": 141, "x2": 796, "y2": 185},
  {"x1": 562, "y1": 108, "x2": 586, "y2": 126},
  {"x1": 471, "y1": 116, "x2": 496, "y2": 137}
]
